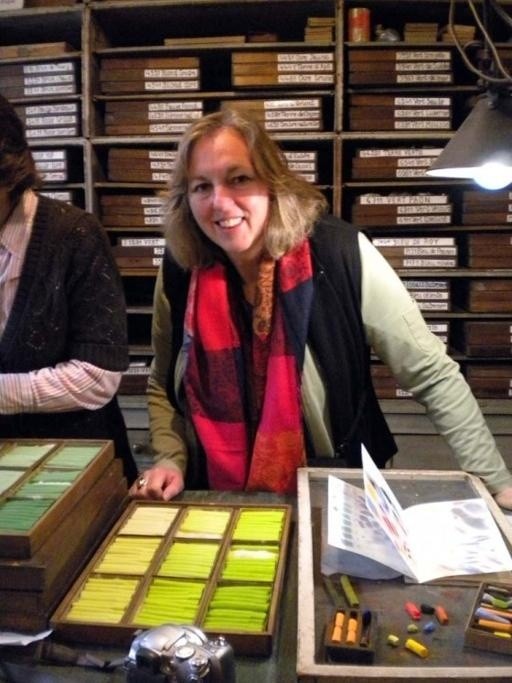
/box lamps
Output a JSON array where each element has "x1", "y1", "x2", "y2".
[{"x1": 426, "y1": 87, "x2": 512, "y2": 191}]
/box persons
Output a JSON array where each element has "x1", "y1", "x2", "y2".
[
  {"x1": 126, "y1": 109, "x2": 512, "y2": 509},
  {"x1": 1, "y1": 91, "x2": 138, "y2": 489}
]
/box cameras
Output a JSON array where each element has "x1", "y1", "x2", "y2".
[{"x1": 123, "y1": 623, "x2": 237, "y2": 683}]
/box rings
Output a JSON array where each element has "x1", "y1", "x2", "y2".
[{"x1": 137, "y1": 478, "x2": 147, "y2": 487}]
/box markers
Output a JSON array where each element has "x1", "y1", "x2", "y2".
[
  {"x1": 387, "y1": 601, "x2": 448, "y2": 658},
  {"x1": 472, "y1": 586, "x2": 512, "y2": 638},
  {"x1": 321, "y1": 574, "x2": 372, "y2": 647}
]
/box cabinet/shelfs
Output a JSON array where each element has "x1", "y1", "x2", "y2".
[{"x1": 0, "y1": 0, "x2": 512, "y2": 400}]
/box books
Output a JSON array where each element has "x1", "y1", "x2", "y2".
[{"x1": 319, "y1": 440, "x2": 512, "y2": 587}]
